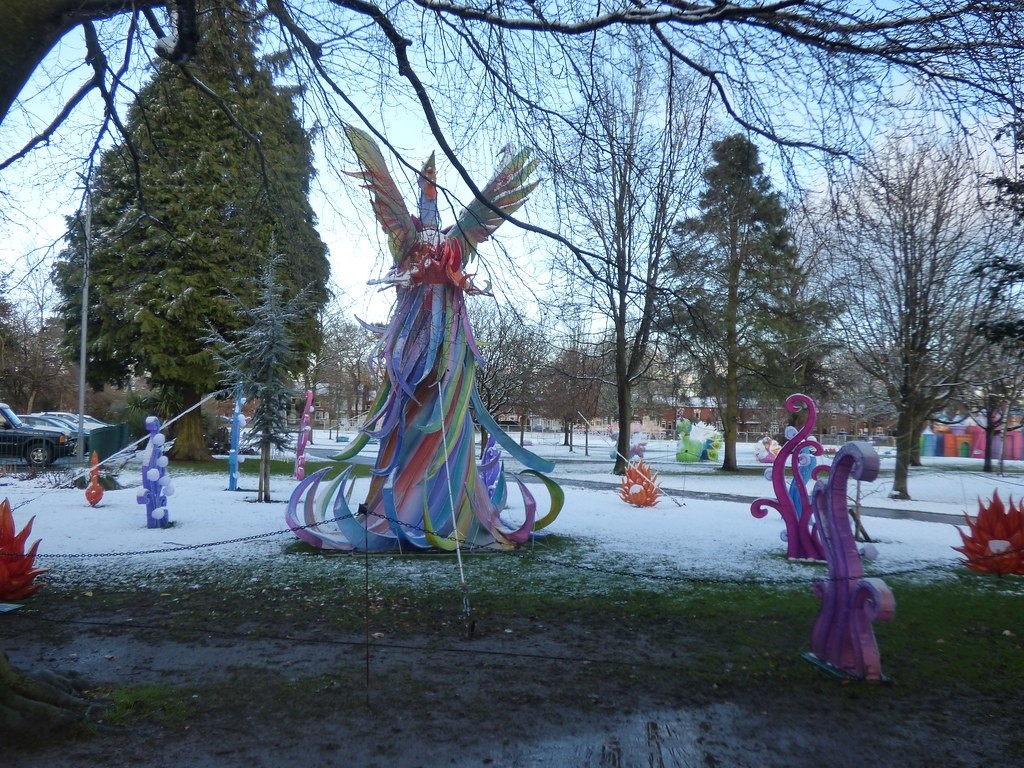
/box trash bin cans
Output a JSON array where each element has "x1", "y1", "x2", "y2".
[{"x1": 88, "y1": 420, "x2": 129, "y2": 466}]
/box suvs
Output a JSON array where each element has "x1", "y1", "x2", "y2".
[{"x1": 0, "y1": 403, "x2": 71, "y2": 468}]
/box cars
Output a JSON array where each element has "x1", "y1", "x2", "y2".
[{"x1": 17, "y1": 411, "x2": 117, "y2": 457}]
